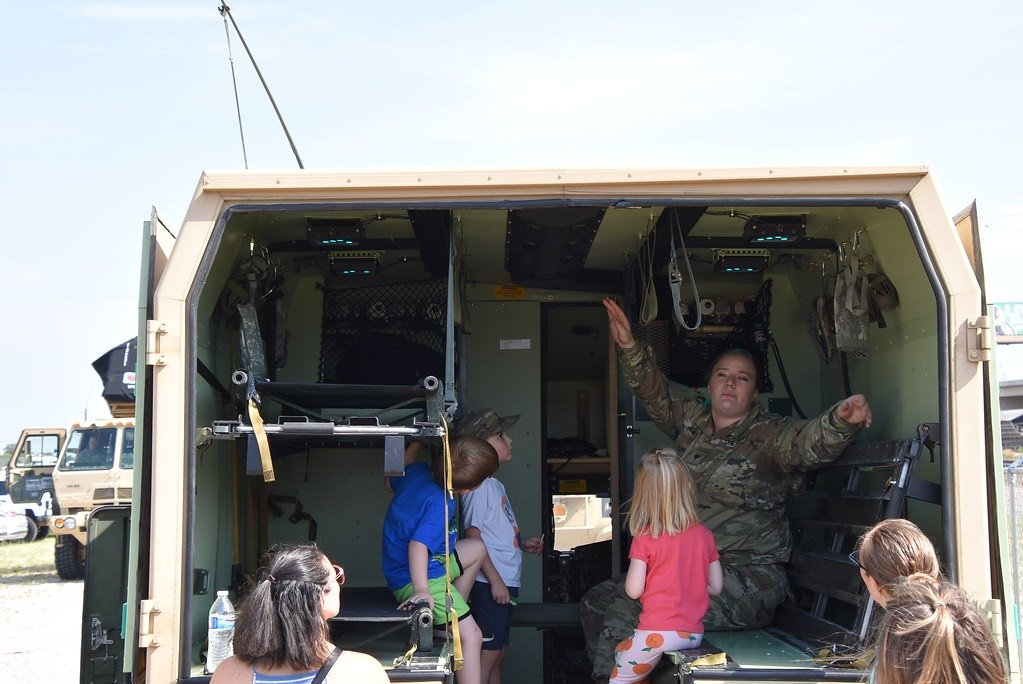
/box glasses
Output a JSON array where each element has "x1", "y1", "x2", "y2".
[
  {"x1": 322, "y1": 564, "x2": 345, "y2": 594},
  {"x1": 849, "y1": 552, "x2": 867, "y2": 575}
]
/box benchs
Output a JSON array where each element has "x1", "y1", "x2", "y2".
[{"x1": 664, "y1": 436, "x2": 922, "y2": 684}]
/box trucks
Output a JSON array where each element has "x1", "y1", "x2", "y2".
[
  {"x1": 72, "y1": 163, "x2": 1023, "y2": 684},
  {"x1": 8, "y1": 413, "x2": 136, "y2": 581}
]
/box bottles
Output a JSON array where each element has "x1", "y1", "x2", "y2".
[{"x1": 207, "y1": 590, "x2": 236, "y2": 673}]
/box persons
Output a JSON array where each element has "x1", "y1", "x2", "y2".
[
  {"x1": 209, "y1": 546, "x2": 391, "y2": 684},
  {"x1": 382, "y1": 436, "x2": 500, "y2": 684},
  {"x1": 850, "y1": 517, "x2": 1005, "y2": 684},
  {"x1": 76, "y1": 436, "x2": 106, "y2": 463},
  {"x1": 579, "y1": 296, "x2": 872, "y2": 684},
  {"x1": 457, "y1": 410, "x2": 545, "y2": 684},
  {"x1": 608, "y1": 448, "x2": 723, "y2": 684}
]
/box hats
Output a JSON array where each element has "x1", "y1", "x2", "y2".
[{"x1": 457, "y1": 408, "x2": 521, "y2": 440}]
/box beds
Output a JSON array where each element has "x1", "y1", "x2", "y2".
[{"x1": 204, "y1": 366, "x2": 457, "y2": 684}]
